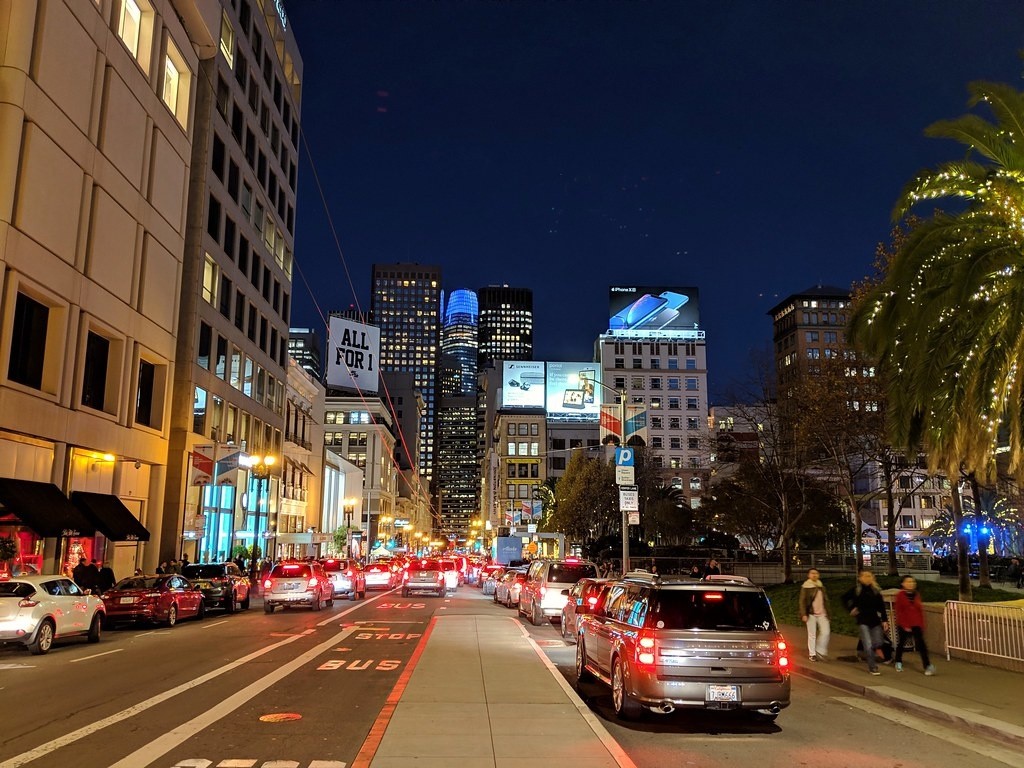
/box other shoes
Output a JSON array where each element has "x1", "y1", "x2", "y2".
[
  {"x1": 868, "y1": 665, "x2": 881, "y2": 675},
  {"x1": 894, "y1": 662, "x2": 903, "y2": 672},
  {"x1": 816, "y1": 651, "x2": 824, "y2": 659},
  {"x1": 925, "y1": 664, "x2": 936, "y2": 676},
  {"x1": 809, "y1": 655, "x2": 818, "y2": 662}
]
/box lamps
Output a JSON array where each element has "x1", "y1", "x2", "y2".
[{"x1": 311, "y1": 526, "x2": 316, "y2": 533}]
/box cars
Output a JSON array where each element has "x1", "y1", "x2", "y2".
[
  {"x1": 101, "y1": 573, "x2": 207, "y2": 627},
  {"x1": 362, "y1": 556, "x2": 409, "y2": 591},
  {"x1": 436, "y1": 557, "x2": 476, "y2": 592},
  {"x1": 471, "y1": 562, "x2": 527, "y2": 609},
  {"x1": 560, "y1": 577, "x2": 614, "y2": 644}
]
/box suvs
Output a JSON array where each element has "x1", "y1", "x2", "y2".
[
  {"x1": 0, "y1": 574, "x2": 107, "y2": 655},
  {"x1": 519, "y1": 559, "x2": 601, "y2": 626},
  {"x1": 180, "y1": 563, "x2": 251, "y2": 614},
  {"x1": 264, "y1": 558, "x2": 367, "y2": 614},
  {"x1": 401, "y1": 559, "x2": 446, "y2": 599},
  {"x1": 575, "y1": 568, "x2": 790, "y2": 718}
]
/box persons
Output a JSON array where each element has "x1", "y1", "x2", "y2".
[
  {"x1": 704, "y1": 558, "x2": 720, "y2": 580},
  {"x1": 583, "y1": 379, "x2": 593, "y2": 396},
  {"x1": 798, "y1": 568, "x2": 832, "y2": 662},
  {"x1": 256, "y1": 556, "x2": 276, "y2": 579},
  {"x1": 156, "y1": 554, "x2": 190, "y2": 574},
  {"x1": 650, "y1": 565, "x2": 657, "y2": 574},
  {"x1": 1009, "y1": 558, "x2": 1024, "y2": 588},
  {"x1": 844, "y1": 568, "x2": 889, "y2": 675},
  {"x1": 234, "y1": 554, "x2": 244, "y2": 571},
  {"x1": 73, "y1": 558, "x2": 116, "y2": 594},
  {"x1": 894, "y1": 576, "x2": 936, "y2": 675},
  {"x1": 601, "y1": 562, "x2": 615, "y2": 577},
  {"x1": 690, "y1": 564, "x2": 702, "y2": 578}
]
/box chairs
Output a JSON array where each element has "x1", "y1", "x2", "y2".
[{"x1": 137, "y1": 579, "x2": 144, "y2": 588}]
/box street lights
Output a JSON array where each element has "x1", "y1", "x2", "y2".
[
  {"x1": 568, "y1": 374, "x2": 630, "y2": 574},
  {"x1": 249, "y1": 454, "x2": 274, "y2": 594},
  {"x1": 467, "y1": 521, "x2": 482, "y2": 553},
  {"x1": 343, "y1": 496, "x2": 356, "y2": 558},
  {"x1": 382, "y1": 516, "x2": 392, "y2": 549},
  {"x1": 404, "y1": 524, "x2": 413, "y2": 555},
  {"x1": 414, "y1": 532, "x2": 422, "y2": 555}
]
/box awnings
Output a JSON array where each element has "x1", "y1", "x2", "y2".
[
  {"x1": 0, "y1": 478, "x2": 96, "y2": 539},
  {"x1": 71, "y1": 491, "x2": 151, "y2": 542}
]
